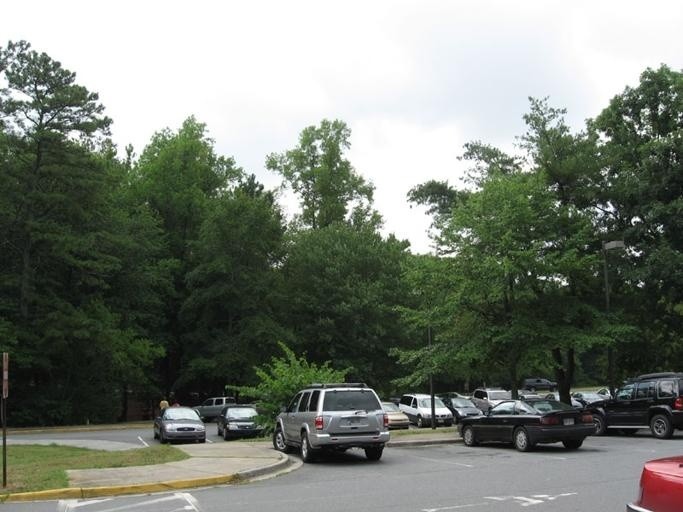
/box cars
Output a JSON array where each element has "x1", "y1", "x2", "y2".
[
  {"x1": 153, "y1": 405, "x2": 206, "y2": 444},
  {"x1": 628, "y1": 458, "x2": 683, "y2": 512},
  {"x1": 378, "y1": 377, "x2": 643, "y2": 437},
  {"x1": 216, "y1": 404, "x2": 264, "y2": 440},
  {"x1": 456, "y1": 396, "x2": 598, "y2": 452}
]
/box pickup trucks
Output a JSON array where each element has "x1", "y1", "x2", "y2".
[{"x1": 192, "y1": 397, "x2": 260, "y2": 422}]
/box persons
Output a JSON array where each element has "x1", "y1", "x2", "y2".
[
  {"x1": 159, "y1": 396, "x2": 168, "y2": 416},
  {"x1": 171, "y1": 399, "x2": 181, "y2": 407}
]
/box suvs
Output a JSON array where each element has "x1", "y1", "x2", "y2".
[
  {"x1": 586, "y1": 371, "x2": 682, "y2": 439},
  {"x1": 271, "y1": 382, "x2": 391, "y2": 462}
]
